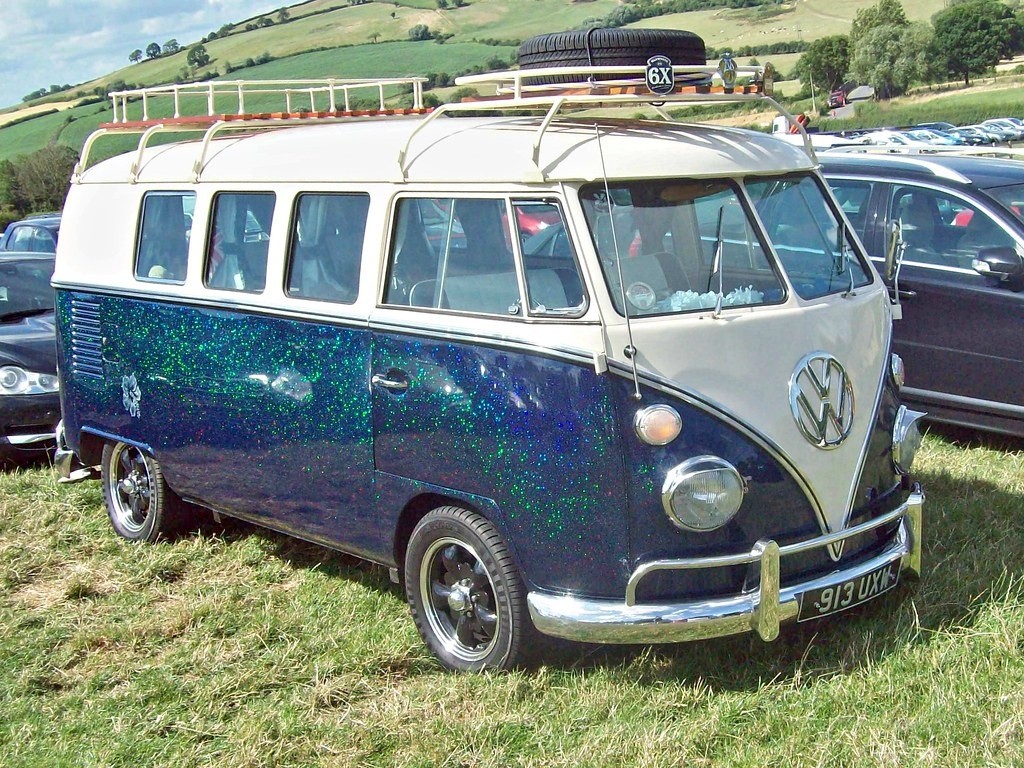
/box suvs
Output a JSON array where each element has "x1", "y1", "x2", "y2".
[
  {"x1": 0, "y1": 213, "x2": 62, "y2": 254},
  {"x1": 626, "y1": 144, "x2": 1024, "y2": 442}
]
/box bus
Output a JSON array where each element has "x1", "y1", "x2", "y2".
[{"x1": 55, "y1": 28, "x2": 923, "y2": 680}]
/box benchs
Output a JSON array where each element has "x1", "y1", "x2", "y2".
[
  {"x1": 404, "y1": 266, "x2": 584, "y2": 314},
  {"x1": 604, "y1": 251, "x2": 691, "y2": 314}
]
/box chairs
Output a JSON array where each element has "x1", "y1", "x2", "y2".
[
  {"x1": 776, "y1": 186, "x2": 827, "y2": 249},
  {"x1": 955, "y1": 202, "x2": 1016, "y2": 269},
  {"x1": 900, "y1": 202, "x2": 947, "y2": 266}
]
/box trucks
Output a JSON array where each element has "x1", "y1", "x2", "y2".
[{"x1": 828, "y1": 90, "x2": 848, "y2": 106}]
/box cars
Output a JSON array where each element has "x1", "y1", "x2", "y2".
[
  {"x1": 0, "y1": 251, "x2": 56, "y2": 468},
  {"x1": 841, "y1": 116, "x2": 1024, "y2": 153}
]
